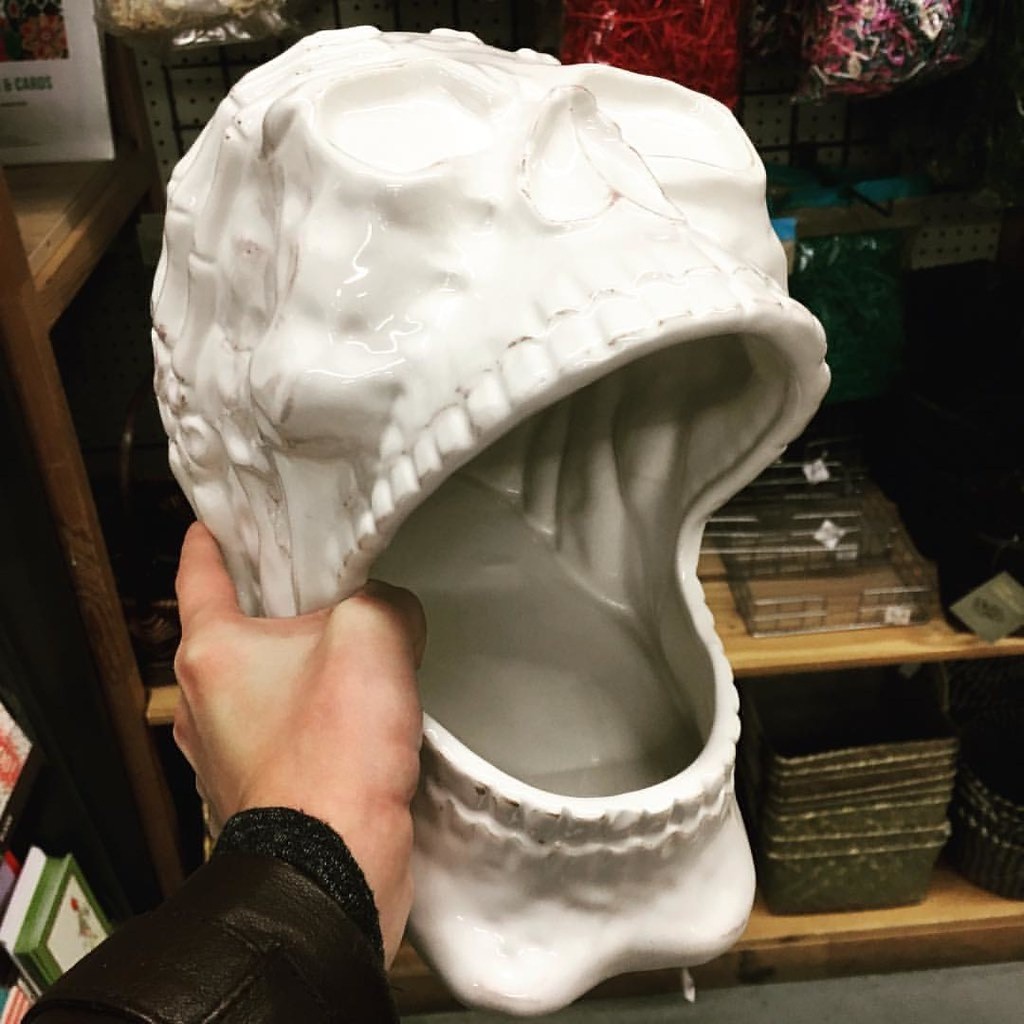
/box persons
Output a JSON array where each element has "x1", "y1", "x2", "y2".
[{"x1": 19, "y1": 518, "x2": 428, "y2": 1024}]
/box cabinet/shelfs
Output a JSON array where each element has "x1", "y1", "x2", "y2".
[{"x1": 1, "y1": 157, "x2": 1023, "y2": 983}]
